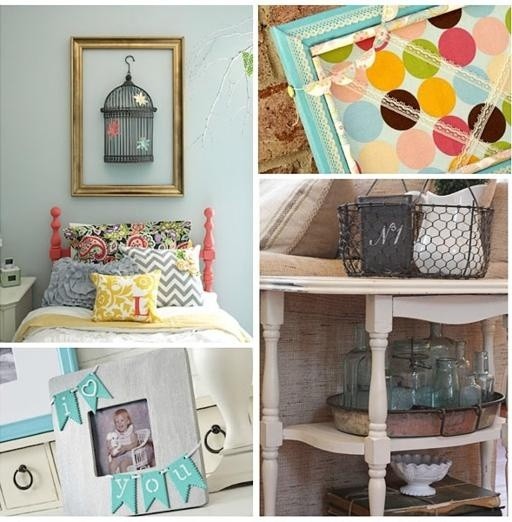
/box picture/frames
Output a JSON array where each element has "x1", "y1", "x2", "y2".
[
  {"x1": 71, "y1": 37, "x2": 185, "y2": 196},
  {"x1": 269, "y1": 5, "x2": 511, "y2": 173},
  {"x1": 48, "y1": 348, "x2": 208, "y2": 516},
  {"x1": 0, "y1": 347, "x2": 78, "y2": 444}
]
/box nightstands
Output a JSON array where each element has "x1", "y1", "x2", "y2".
[{"x1": 0, "y1": 277, "x2": 38, "y2": 340}]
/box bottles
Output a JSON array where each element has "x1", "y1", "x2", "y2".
[
  {"x1": 342, "y1": 321, "x2": 373, "y2": 411},
  {"x1": 389, "y1": 338, "x2": 435, "y2": 411},
  {"x1": 432, "y1": 350, "x2": 494, "y2": 410}
]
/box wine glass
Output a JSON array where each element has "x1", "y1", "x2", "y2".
[{"x1": 390, "y1": 454, "x2": 453, "y2": 497}]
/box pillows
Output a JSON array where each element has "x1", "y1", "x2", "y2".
[
  {"x1": 118, "y1": 241, "x2": 205, "y2": 308},
  {"x1": 39, "y1": 255, "x2": 144, "y2": 315},
  {"x1": 88, "y1": 269, "x2": 161, "y2": 323},
  {"x1": 63, "y1": 221, "x2": 192, "y2": 262},
  {"x1": 260, "y1": 174, "x2": 357, "y2": 258}
]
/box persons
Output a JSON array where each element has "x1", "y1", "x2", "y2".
[{"x1": 108, "y1": 408, "x2": 139, "y2": 475}]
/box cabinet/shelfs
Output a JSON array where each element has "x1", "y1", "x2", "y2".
[
  {"x1": 1, "y1": 348, "x2": 251, "y2": 517},
  {"x1": 260, "y1": 277, "x2": 507, "y2": 516}
]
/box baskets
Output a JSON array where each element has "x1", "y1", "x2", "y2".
[{"x1": 338, "y1": 179, "x2": 494, "y2": 279}]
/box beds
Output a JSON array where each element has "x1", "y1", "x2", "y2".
[{"x1": 13, "y1": 207, "x2": 253, "y2": 347}]
